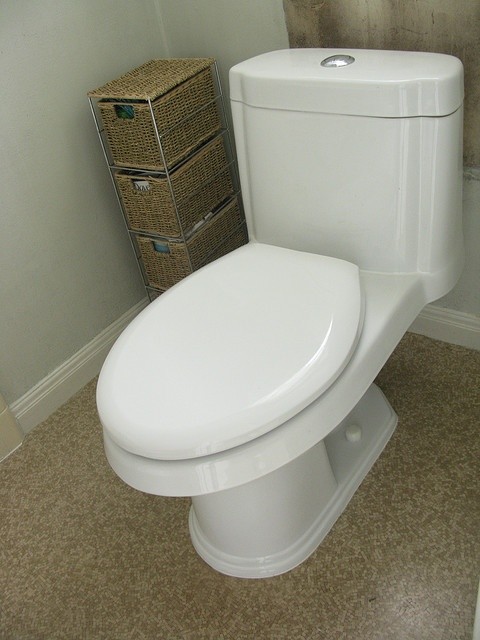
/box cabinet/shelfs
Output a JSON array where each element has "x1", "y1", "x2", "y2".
[{"x1": 85, "y1": 58, "x2": 249, "y2": 303}]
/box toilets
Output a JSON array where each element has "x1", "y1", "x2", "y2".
[{"x1": 94, "y1": 42, "x2": 466, "y2": 580}]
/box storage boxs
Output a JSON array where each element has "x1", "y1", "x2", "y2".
[{"x1": 145, "y1": 285, "x2": 164, "y2": 304}]
